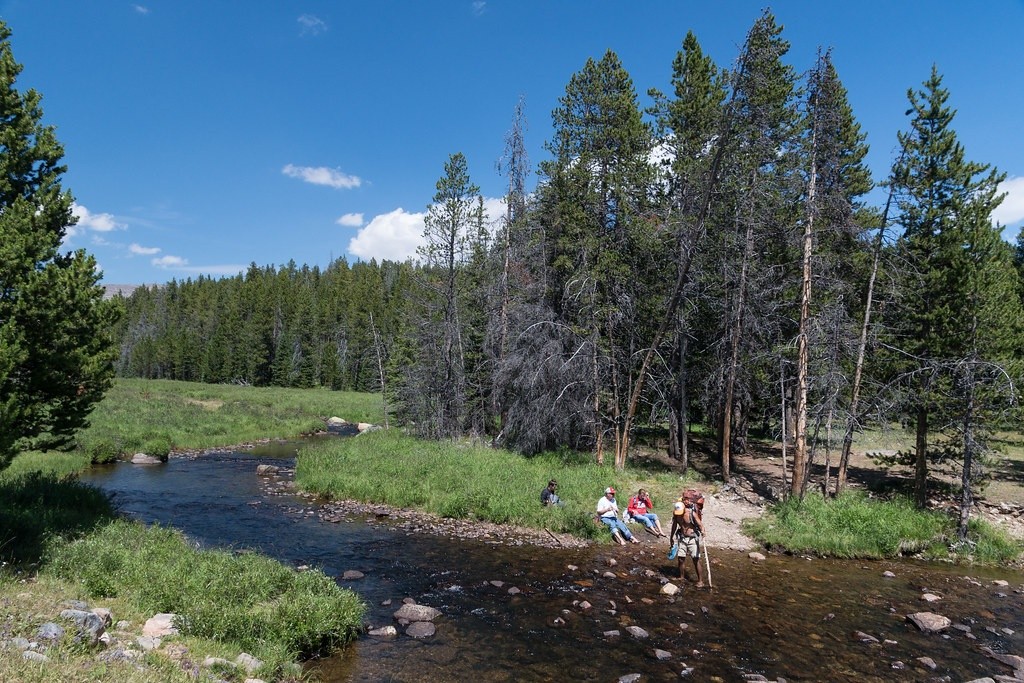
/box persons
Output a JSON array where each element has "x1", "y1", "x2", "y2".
[
  {"x1": 541, "y1": 479, "x2": 563, "y2": 506},
  {"x1": 670, "y1": 502, "x2": 706, "y2": 588},
  {"x1": 597, "y1": 487, "x2": 641, "y2": 546},
  {"x1": 628, "y1": 489, "x2": 667, "y2": 537}
]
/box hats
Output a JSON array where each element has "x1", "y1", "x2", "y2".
[
  {"x1": 674, "y1": 501, "x2": 685, "y2": 515},
  {"x1": 603, "y1": 486, "x2": 616, "y2": 494}
]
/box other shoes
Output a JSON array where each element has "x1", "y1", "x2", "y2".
[{"x1": 666, "y1": 543, "x2": 678, "y2": 560}]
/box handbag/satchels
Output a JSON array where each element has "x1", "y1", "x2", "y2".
[{"x1": 673, "y1": 489, "x2": 706, "y2": 539}]
